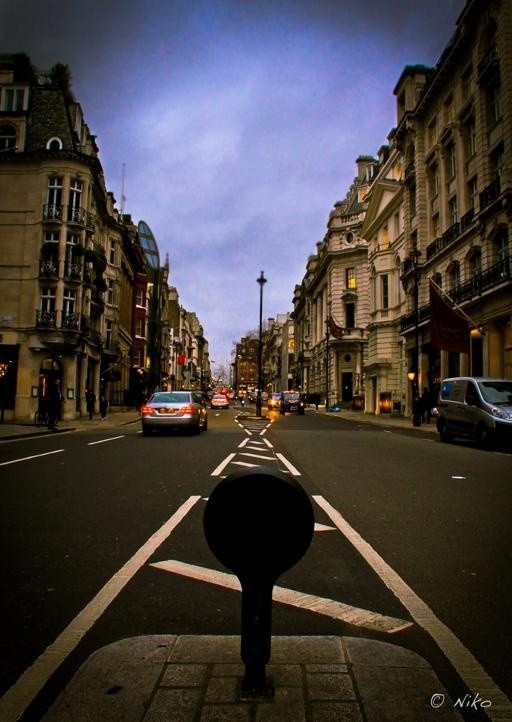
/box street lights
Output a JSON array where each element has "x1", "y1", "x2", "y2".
[
  {"x1": 408, "y1": 246, "x2": 422, "y2": 427},
  {"x1": 255, "y1": 268, "x2": 267, "y2": 416}
]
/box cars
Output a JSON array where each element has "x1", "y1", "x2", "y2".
[
  {"x1": 210, "y1": 394, "x2": 229, "y2": 409},
  {"x1": 267, "y1": 390, "x2": 305, "y2": 415},
  {"x1": 140, "y1": 391, "x2": 208, "y2": 435}
]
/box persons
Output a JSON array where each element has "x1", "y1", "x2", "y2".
[
  {"x1": 48, "y1": 379, "x2": 66, "y2": 430},
  {"x1": 100, "y1": 395, "x2": 108, "y2": 421},
  {"x1": 86, "y1": 391, "x2": 96, "y2": 420},
  {"x1": 421, "y1": 387, "x2": 432, "y2": 424}
]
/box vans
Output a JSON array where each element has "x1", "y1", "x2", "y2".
[{"x1": 436, "y1": 376, "x2": 512, "y2": 445}]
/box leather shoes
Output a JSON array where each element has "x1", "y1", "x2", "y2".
[{"x1": 48, "y1": 424, "x2": 59, "y2": 431}]
